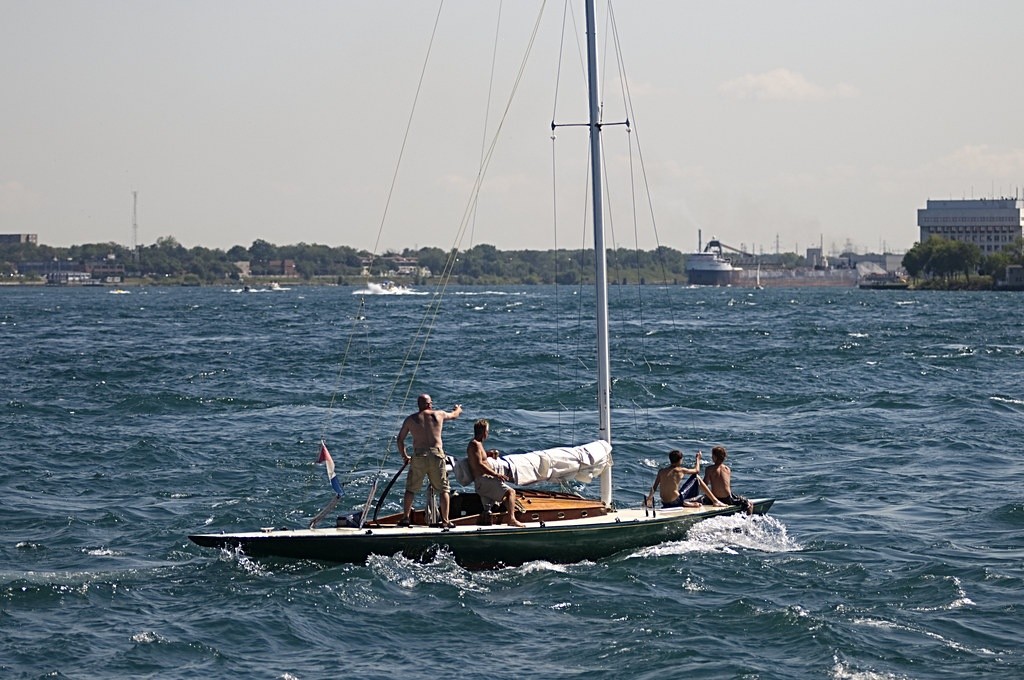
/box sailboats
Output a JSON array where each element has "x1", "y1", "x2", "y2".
[{"x1": 184, "y1": 0, "x2": 775, "y2": 562}]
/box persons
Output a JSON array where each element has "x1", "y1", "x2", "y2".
[
  {"x1": 698, "y1": 447, "x2": 753, "y2": 515},
  {"x1": 398, "y1": 393, "x2": 464, "y2": 528},
  {"x1": 645, "y1": 449, "x2": 729, "y2": 508},
  {"x1": 467, "y1": 419, "x2": 528, "y2": 528}
]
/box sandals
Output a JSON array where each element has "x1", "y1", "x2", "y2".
[
  {"x1": 442, "y1": 521, "x2": 455, "y2": 528},
  {"x1": 397, "y1": 517, "x2": 410, "y2": 526}
]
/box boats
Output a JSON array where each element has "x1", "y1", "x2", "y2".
[
  {"x1": 858, "y1": 273, "x2": 910, "y2": 289},
  {"x1": 681, "y1": 251, "x2": 744, "y2": 286}
]
